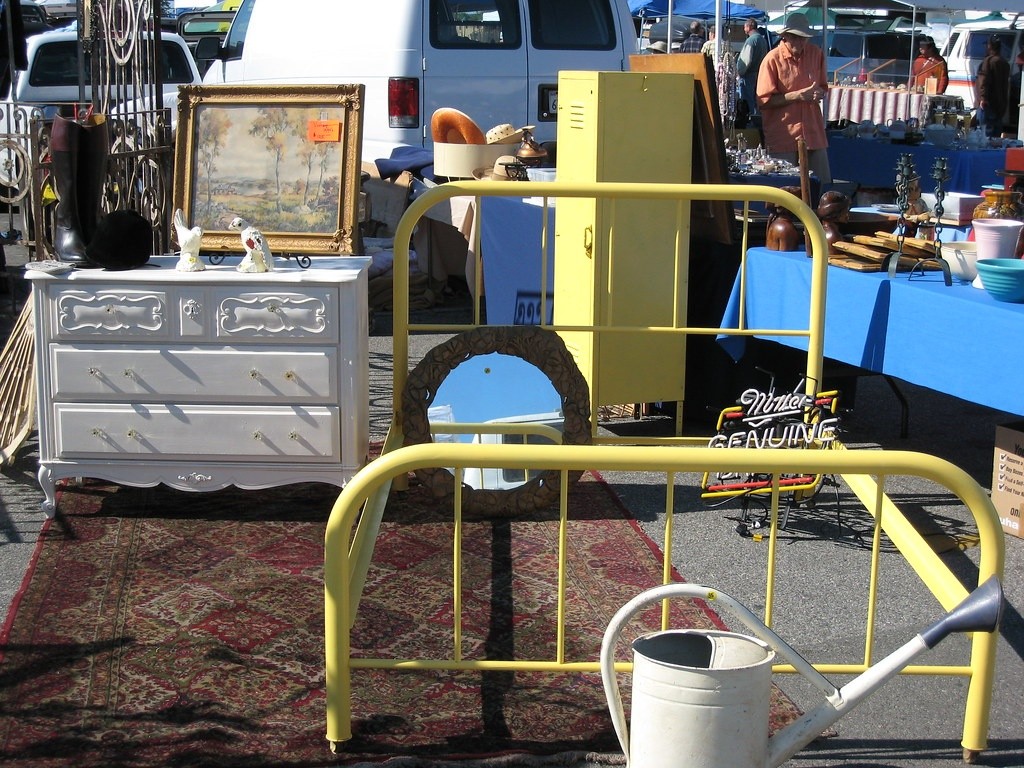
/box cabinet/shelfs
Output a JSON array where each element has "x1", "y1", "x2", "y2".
[
  {"x1": 24, "y1": 254, "x2": 374, "y2": 519},
  {"x1": 549, "y1": 70, "x2": 695, "y2": 445}
]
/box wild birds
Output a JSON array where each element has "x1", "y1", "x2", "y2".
[
  {"x1": 227, "y1": 217, "x2": 274, "y2": 271},
  {"x1": 173, "y1": 208, "x2": 204, "y2": 259}
]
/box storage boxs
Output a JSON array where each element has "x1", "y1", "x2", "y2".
[
  {"x1": 989, "y1": 422, "x2": 1024, "y2": 540},
  {"x1": 921, "y1": 191, "x2": 986, "y2": 222}
]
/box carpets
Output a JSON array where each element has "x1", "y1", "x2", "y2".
[{"x1": 0, "y1": 441, "x2": 840, "y2": 768}]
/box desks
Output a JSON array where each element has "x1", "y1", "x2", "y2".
[
  {"x1": 715, "y1": 242, "x2": 1024, "y2": 419},
  {"x1": 725, "y1": 164, "x2": 821, "y2": 218},
  {"x1": 416, "y1": 167, "x2": 558, "y2": 330},
  {"x1": 827, "y1": 126, "x2": 1007, "y2": 196}
]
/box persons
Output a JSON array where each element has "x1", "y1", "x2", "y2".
[
  {"x1": 679, "y1": 21, "x2": 706, "y2": 54},
  {"x1": 817, "y1": 191, "x2": 851, "y2": 256},
  {"x1": 908, "y1": 36, "x2": 949, "y2": 94},
  {"x1": 756, "y1": 16, "x2": 831, "y2": 182},
  {"x1": 971, "y1": 36, "x2": 1011, "y2": 139},
  {"x1": 736, "y1": 19, "x2": 767, "y2": 127},
  {"x1": 893, "y1": 171, "x2": 936, "y2": 238},
  {"x1": 701, "y1": 26, "x2": 732, "y2": 67},
  {"x1": 765, "y1": 186, "x2": 802, "y2": 252}
]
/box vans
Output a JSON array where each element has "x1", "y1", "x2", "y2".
[
  {"x1": 194, "y1": 0, "x2": 639, "y2": 238},
  {"x1": 0, "y1": 0, "x2": 1024, "y2": 215},
  {"x1": 453, "y1": 411, "x2": 564, "y2": 490}
]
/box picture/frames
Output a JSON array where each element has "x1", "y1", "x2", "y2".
[
  {"x1": 170, "y1": 84, "x2": 366, "y2": 268},
  {"x1": 828, "y1": 231, "x2": 945, "y2": 273}
]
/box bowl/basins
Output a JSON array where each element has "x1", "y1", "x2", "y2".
[
  {"x1": 940, "y1": 241, "x2": 978, "y2": 281},
  {"x1": 926, "y1": 124, "x2": 956, "y2": 146},
  {"x1": 974, "y1": 258, "x2": 1024, "y2": 303}
]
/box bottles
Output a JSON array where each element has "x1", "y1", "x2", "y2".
[
  {"x1": 972, "y1": 190, "x2": 1024, "y2": 289},
  {"x1": 966, "y1": 126, "x2": 983, "y2": 150}
]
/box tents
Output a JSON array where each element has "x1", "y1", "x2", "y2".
[{"x1": 627, "y1": 0, "x2": 770, "y2": 53}]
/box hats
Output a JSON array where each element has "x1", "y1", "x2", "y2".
[
  {"x1": 486, "y1": 124, "x2": 535, "y2": 144},
  {"x1": 777, "y1": 13, "x2": 816, "y2": 37},
  {"x1": 646, "y1": 41, "x2": 667, "y2": 53},
  {"x1": 982, "y1": 32, "x2": 1002, "y2": 44},
  {"x1": 471, "y1": 156, "x2": 526, "y2": 181}
]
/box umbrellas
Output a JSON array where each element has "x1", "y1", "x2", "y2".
[{"x1": 768, "y1": 2, "x2": 864, "y2": 29}]
